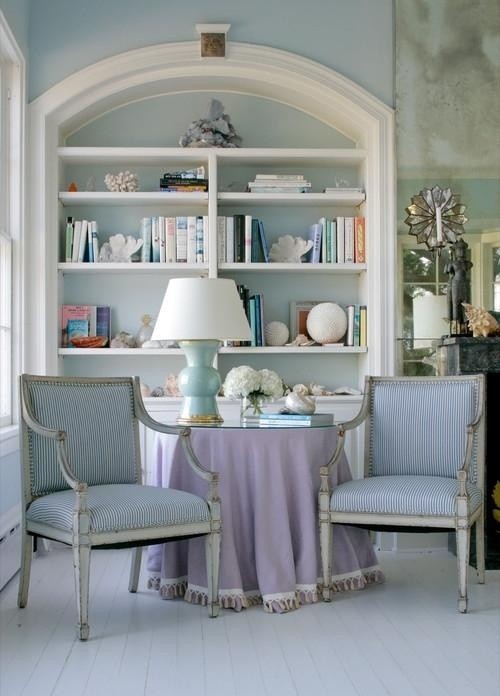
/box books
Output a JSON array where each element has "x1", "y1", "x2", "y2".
[
  {"x1": 259, "y1": 419, "x2": 333, "y2": 426},
  {"x1": 307, "y1": 216, "x2": 365, "y2": 264},
  {"x1": 323, "y1": 188, "x2": 365, "y2": 193},
  {"x1": 219, "y1": 285, "x2": 266, "y2": 347},
  {"x1": 247, "y1": 175, "x2": 313, "y2": 193},
  {"x1": 217, "y1": 215, "x2": 270, "y2": 263},
  {"x1": 344, "y1": 305, "x2": 366, "y2": 347},
  {"x1": 160, "y1": 165, "x2": 208, "y2": 193},
  {"x1": 242, "y1": 415, "x2": 259, "y2": 419},
  {"x1": 65, "y1": 216, "x2": 99, "y2": 263},
  {"x1": 138, "y1": 216, "x2": 209, "y2": 263},
  {"x1": 240, "y1": 418, "x2": 259, "y2": 423},
  {"x1": 61, "y1": 305, "x2": 111, "y2": 348},
  {"x1": 259, "y1": 411, "x2": 334, "y2": 421}
]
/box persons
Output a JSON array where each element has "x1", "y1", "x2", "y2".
[
  {"x1": 164, "y1": 374, "x2": 178, "y2": 394},
  {"x1": 443, "y1": 251, "x2": 453, "y2": 320},
  {"x1": 448, "y1": 238, "x2": 474, "y2": 323},
  {"x1": 135, "y1": 314, "x2": 153, "y2": 347}
]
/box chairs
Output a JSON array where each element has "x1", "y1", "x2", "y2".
[
  {"x1": 318, "y1": 375, "x2": 488, "y2": 613},
  {"x1": 18, "y1": 375, "x2": 223, "y2": 641}
]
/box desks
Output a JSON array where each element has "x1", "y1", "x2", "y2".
[
  {"x1": 434, "y1": 337, "x2": 500, "y2": 374},
  {"x1": 147, "y1": 424, "x2": 385, "y2": 616}
]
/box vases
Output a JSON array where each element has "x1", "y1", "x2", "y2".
[{"x1": 242, "y1": 397, "x2": 269, "y2": 419}]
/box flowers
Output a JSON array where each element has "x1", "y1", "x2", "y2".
[{"x1": 222, "y1": 365, "x2": 285, "y2": 412}]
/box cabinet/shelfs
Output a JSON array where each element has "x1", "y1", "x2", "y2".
[{"x1": 59, "y1": 148, "x2": 375, "y2": 486}]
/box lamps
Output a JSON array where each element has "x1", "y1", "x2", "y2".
[{"x1": 150, "y1": 277, "x2": 255, "y2": 423}]
